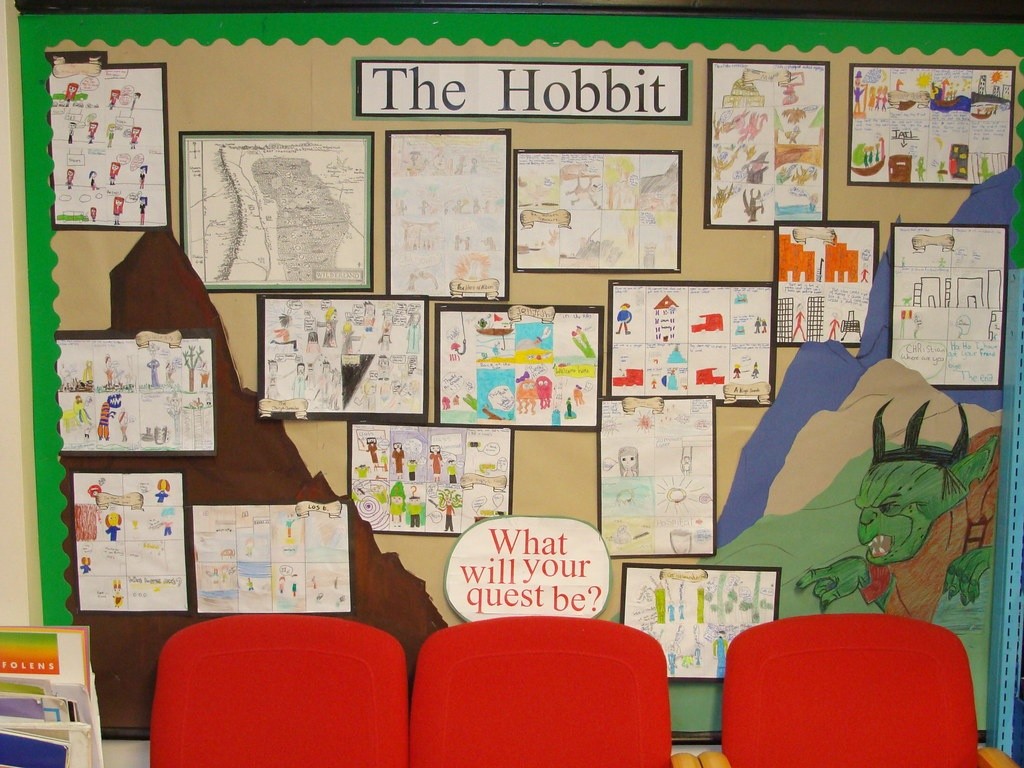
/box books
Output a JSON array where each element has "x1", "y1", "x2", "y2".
[{"x1": 0, "y1": 626, "x2": 104, "y2": 768}]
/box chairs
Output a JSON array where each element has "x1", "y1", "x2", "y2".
[
  {"x1": 150, "y1": 614, "x2": 409, "y2": 768},
  {"x1": 408, "y1": 617, "x2": 702, "y2": 768},
  {"x1": 696, "y1": 614, "x2": 1019, "y2": 768}
]
